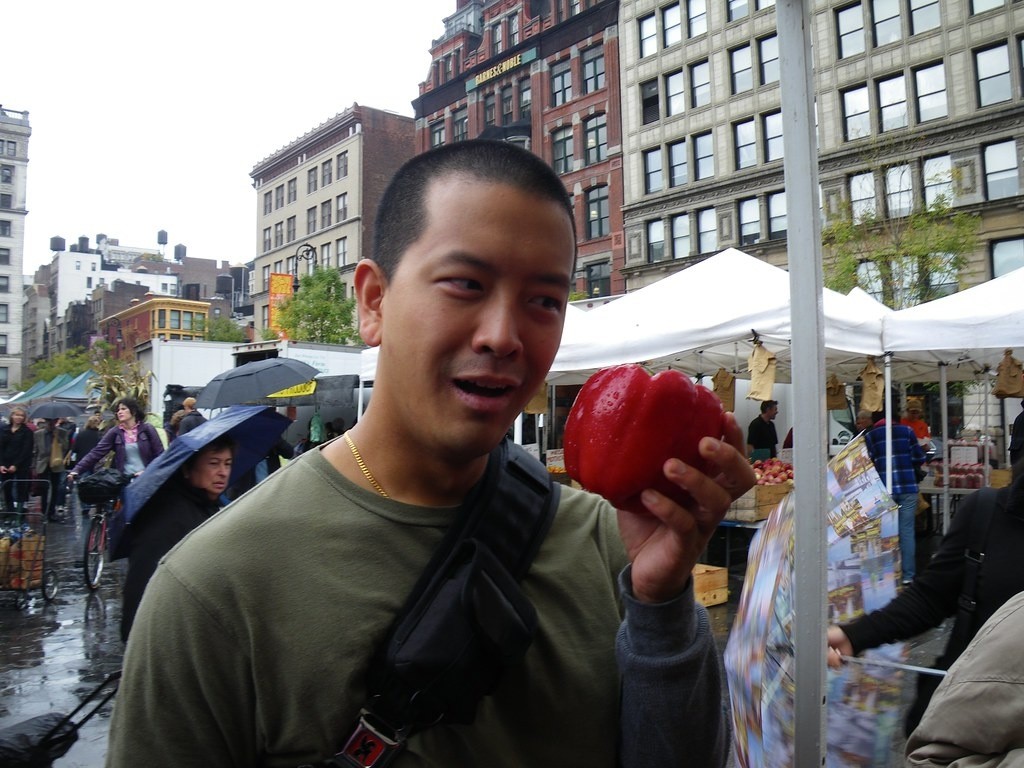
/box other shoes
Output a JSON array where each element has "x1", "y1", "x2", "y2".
[
  {"x1": 82, "y1": 510, "x2": 89, "y2": 515},
  {"x1": 902, "y1": 579, "x2": 913, "y2": 584}
]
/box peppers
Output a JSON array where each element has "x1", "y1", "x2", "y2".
[{"x1": 564, "y1": 364, "x2": 725, "y2": 517}]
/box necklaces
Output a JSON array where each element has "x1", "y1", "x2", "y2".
[{"x1": 343, "y1": 430, "x2": 391, "y2": 498}]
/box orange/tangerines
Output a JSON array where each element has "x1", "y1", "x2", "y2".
[{"x1": 547, "y1": 465, "x2": 566, "y2": 473}]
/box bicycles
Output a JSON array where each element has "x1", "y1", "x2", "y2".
[{"x1": 63, "y1": 472, "x2": 138, "y2": 593}]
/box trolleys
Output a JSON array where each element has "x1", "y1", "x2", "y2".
[{"x1": 0, "y1": 478, "x2": 59, "y2": 612}]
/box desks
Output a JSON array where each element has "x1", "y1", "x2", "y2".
[
  {"x1": 917, "y1": 469, "x2": 1011, "y2": 528},
  {"x1": 719, "y1": 520, "x2": 766, "y2": 577}
]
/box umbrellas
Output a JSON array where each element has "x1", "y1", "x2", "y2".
[
  {"x1": 724, "y1": 429, "x2": 947, "y2": 768},
  {"x1": 107, "y1": 405, "x2": 294, "y2": 564},
  {"x1": 27, "y1": 400, "x2": 83, "y2": 420},
  {"x1": 193, "y1": 357, "x2": 322, "y2": 410}
]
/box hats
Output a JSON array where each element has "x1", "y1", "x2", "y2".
[{"x1": 906, "y1": 400, "x2": 922, "y2": 411}]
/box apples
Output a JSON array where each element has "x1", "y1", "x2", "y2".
[{"x1": 747, "y1": 458, "x2": 795, "y2": 486}]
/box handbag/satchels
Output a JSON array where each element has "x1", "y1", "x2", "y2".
[{"x1": 51, "y1": 435, "x2": 65, "y2": 473}]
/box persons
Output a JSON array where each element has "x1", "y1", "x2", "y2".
[
  {"x1": 747, "y1": 400, "x2": 779, "y2": 459},
  {"x1": 178, "y1": 397, "x2": 207, "y2": 437},
  {"x1": 904, "y1": 590, "x2": 1024, "y2": 768},
  {"x1": 900, "y1": 399, "x2": 930, "y2": 449},
  {"x1": 303, "y1": 417, "x2": 345, "y2": 453},
  {"x1": 67, "y1": 399, "x2": 165, "y2": 484},
  {"x1": 0, "y1": 406, "x2": 104, "y2": 522},
  {"x1": 241, "y1": 438, "x2": 295, "y2": 491},
  {"x1": 825, "y1": 457, "x2": 1024, "y2": 739},
  {"x1": 865, "y1": 403, "x2": 925, "y2": 584},
  {"x1": 104, "y1": 141, "x2": 758, "y2": 768},
  {"x1": 856, "y1": 410, "x2": 873, "y2": 429},
  {"x1": 119, "y1": 432, "x2": 235, "y2": 647}
]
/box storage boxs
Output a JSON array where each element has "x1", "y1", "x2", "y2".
[
  {"x1": 692, "y1": 563, "x2": 728, "y2": 607},
  {"x1": 755, "y1": 484, "x2": 790, "y2": 522},
  {"x1": 549, "y1": 472, "x2": 571, "y2": 487},
  {"x1": 0, "y1": 534, "x2": 45, "y2": 591}
]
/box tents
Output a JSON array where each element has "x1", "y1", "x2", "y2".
[
  {"x1": 11, "y1": 368, "x2": 101, "y2": 403},
  {"x1": 357, "y1": 248, "x2": 1024, "y2": 534}
]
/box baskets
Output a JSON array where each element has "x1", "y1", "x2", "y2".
[{"x1": 77, "y1": 483, "x2": 121, "y2": 504}]
